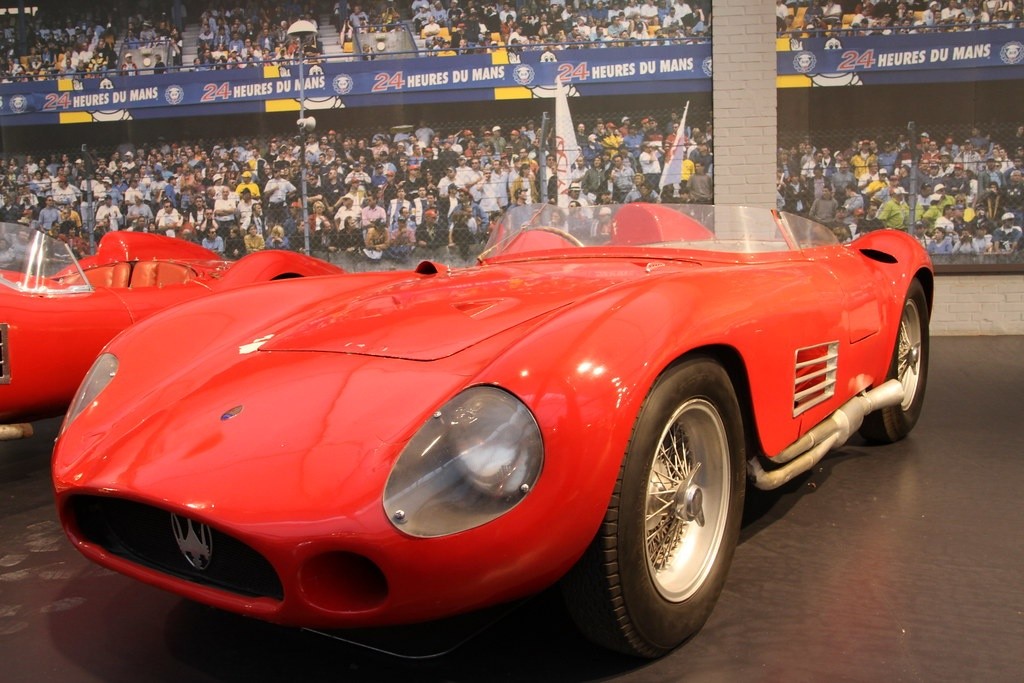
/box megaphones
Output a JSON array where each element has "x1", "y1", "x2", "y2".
[{"x1": 297, "y1": 117, "x2": 316, "y2": 131}]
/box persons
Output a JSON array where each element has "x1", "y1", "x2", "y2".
[
  {"x1": 0, "y1": 111, "x2": 714, "y2": 272},
  {"x1": 777, "y1": 0, "x2": 1024, "y2": 38},
  {"x1": 0, "y1": 0, "x2": 712, "y2": 83},
  {"x1": 777, "y1": 124, "x2": 1024, "y2": 264}
]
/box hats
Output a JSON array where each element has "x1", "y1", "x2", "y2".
[
  {"x1": 776, "y1": 0, "x2": 1024, "y2": 38},
  {"x1": 0, "y1": 111, "x2": 715, "y2": 262},
  {"x1": 777, "y1": 132, "x2": 1024, "y2": 254},
  {"x1": 0, "y1": 0, "x2": 716, "y2": 86}
]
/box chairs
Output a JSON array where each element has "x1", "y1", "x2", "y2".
[
  {"x1": 64, "y1": 261, "x2": 132, "y2": 288},
  {"x1": 20, "y1": 54, "x2": 107, "y2": 78},
  {"x1": 343, "y1": 41, "x2": 353, "y2": 53},
  {"x1": 650, "y1": 25, "x2": 662, "y2": 37},
  {"x1": 421, "y1": 28, "x2": 506, "y2": 55},
  {"x1": 130, "y1": 261, "x2": 197, "y2": 286}
]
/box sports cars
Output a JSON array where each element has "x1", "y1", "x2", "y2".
[
  {"x1": 0, "y1": 217, "x2": 348, "y2": 431},
  {"x1": 52, "y1": 198, "x2": 937, "y2": 670}
]
